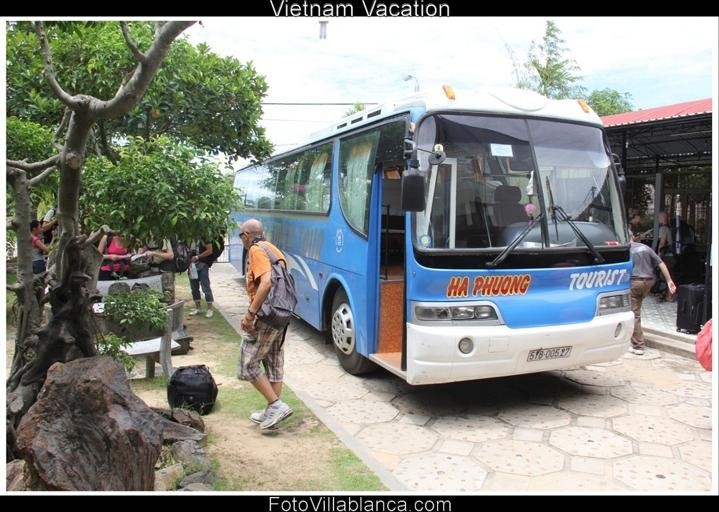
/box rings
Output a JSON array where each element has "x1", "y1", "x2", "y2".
[{"x1": 245, "y1": 328, "x2": 247, "y2": 331}]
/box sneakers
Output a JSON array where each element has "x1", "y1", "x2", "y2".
[
  {"x1": 189, "y1": 307, "x2": 202, "y2": 316},
  {"x1": 628, "y1": 346, "x2": 643, "y2": 355},
  {"x1": 249, "y1": 410, "x2": 280, "y2": 430},
  {"x1": 260, "y1": 401, "x2": 293, "y2": 430},
  {"x1": 205, "y1": 309, "x2": 214, "y2": 317}
]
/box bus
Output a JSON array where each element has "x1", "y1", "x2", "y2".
[{"x1": 222, "y1": 84, "x2": 635, "y2": 388}]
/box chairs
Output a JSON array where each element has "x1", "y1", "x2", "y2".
[
  {"x1": 494, "y1": 186, "x2": 531, "y2": 228},
  {"x1": 377, "y1": 177, "x2": 404, "y2": 248}
]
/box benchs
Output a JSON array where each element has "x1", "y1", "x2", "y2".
[{"x1": 92, "y1": 274, "x2": 193, "y2": 379}]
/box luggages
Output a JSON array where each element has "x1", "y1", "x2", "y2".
[{"x1": 676, "y1": 283, "x2": 705, "y2": 334}]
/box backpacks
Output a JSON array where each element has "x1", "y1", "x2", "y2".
[
  {"x1": 152, "y1": 237, "x2": 193, "y2": 273},
  {"x1": 196, "y1": 234, "x2": 224, "y2": 262}
]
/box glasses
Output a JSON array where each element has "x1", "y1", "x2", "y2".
[{"x1": 239, "y1": 232, "x2": 249, "y2": 238}]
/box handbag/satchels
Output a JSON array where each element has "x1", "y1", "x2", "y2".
[
  {"x1": 167, "y1": 365, "x2": 217, "y2": 415},
  {"x1": 250, "y1": 241, "x2": 298, "y2": 330},
  {"x1": 40, "y1": 208, "x2": 56, "y2": 244},
  {"x1": 695, "y1": 319, "x2": 712, "y2": 371}
]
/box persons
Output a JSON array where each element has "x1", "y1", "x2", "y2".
[
  {"x1": 627, "y1": 208, "x2": 711, "y2": 303},
  {"x1": 627, "y1": 229, "x2": 678, "y2": 355},
  {"x1": 31, "y1": 206, "x2": 225, "y2": 319},
  {"x1": 238, "y1": 219, "x2": 295, "y2": 430}
]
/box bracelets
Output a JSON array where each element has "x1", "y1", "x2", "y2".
[
  {"x1": 247, "y1": 308, "x2": 256, "y2": 316},
  {"x1": 245, "y1": 315, "x2": 254, "y2": 322}
]
